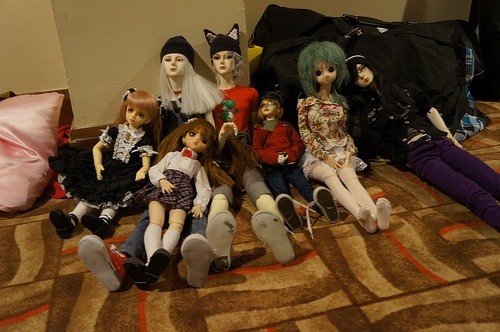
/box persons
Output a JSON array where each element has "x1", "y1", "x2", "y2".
[
  {"x1": 124, "y1": 119, "x2": 219, "y2": 291},
  {"x1": 48, "y1": 88, "x2": 163, "y2": 240},
  {"x1": 76, "y1": 35, "x2": 238, "y2": 291},
  {"x1": 345, "y1": 55, "x2": 500, "y2": 233},
  {"x1": 203, "y1": 23, "x2": 294, "y2": 271},
  {"x1": 253, "y1": 90, "x2": 339, "y2": 231},
  {"x1": 296, "y1": 42, "x2": 391, "y2": 234}
]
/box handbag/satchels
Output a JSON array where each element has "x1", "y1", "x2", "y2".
[{"x1": 249, "y1": 3, "x2": 492, "y2": 145}]
